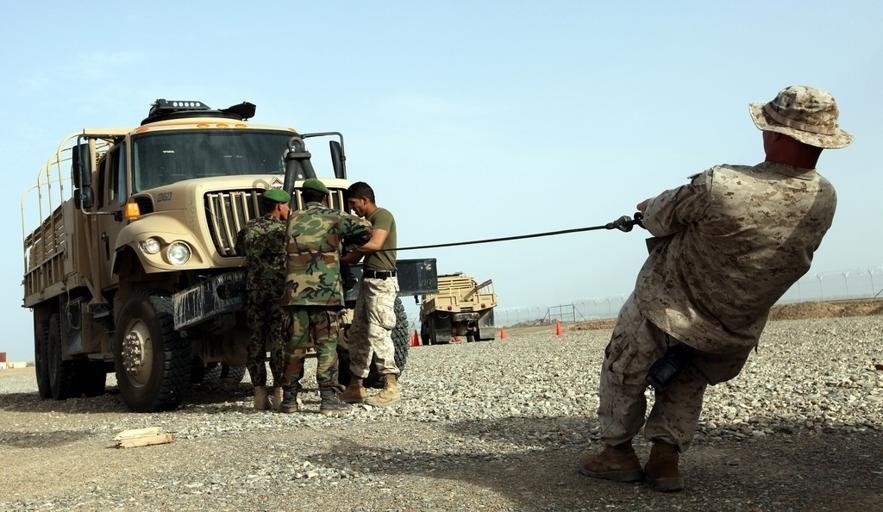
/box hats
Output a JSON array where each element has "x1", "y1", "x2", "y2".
[
  {"x1": 301, "y1": 178, "x2": 331, "y2": 196},
  {"x1": 262, "y1": 188, "x2": 292, "y2": 204},
  {"x1": 745, "y1": 83, "x2": 856, "y2": 151}
]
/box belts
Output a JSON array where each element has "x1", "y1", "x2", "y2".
[{"x1": 362, "y1": 268, "x2": 395, "y2": 281}]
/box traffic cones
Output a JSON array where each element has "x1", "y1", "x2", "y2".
[
  {"x1": 500, "y1": 329, "x2": 506, "y2": 340},
  {"x1": 556, "y1": 320, "x2": 564, "y2": 335},
  {"x1": 410, "y1": 328, "x2": 420, "y2": 346}
]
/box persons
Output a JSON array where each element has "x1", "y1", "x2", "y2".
[
  {"x1": 571, "y1": 82, "x2": 858, "y2": 495},
  {"x1": 280, "y1": 177, "x2": 375, "y2": 414},
  {"x1": 233, "y1": 188, "x2": 292, "y2": 411},
  {"x1": 335, "y1": 181, "x2": 405, "y2": 405}
]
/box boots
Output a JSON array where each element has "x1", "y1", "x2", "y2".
[
  {"x1": 577, "y1": 437, "x2": 643, "y2": 484},
  {"x1": 335, "y1": 379, "x2": 368, "y2": 404},
  {"x1": 641, "y1": 438, "x2": 685, "y2": 492},
  {"x1": 271, "y1": 384, "x2": 302, "y2": 414},
  {"x1": 318, "y1": 384, "x2": 354, "y2": 415},
  {"x1": 363, "y1": 373, "x2": 401, "y2": 407},
  {"x1": 253, "y1": 385, "x2": 272, "y2": 412}
]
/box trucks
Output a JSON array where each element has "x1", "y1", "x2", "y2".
[
  {"x1": 415, "y1": 270, "x2": 498, "y2": 346},
  {"x1": 19, "y1": 95, "x2": 438, "y2": 423}
]
